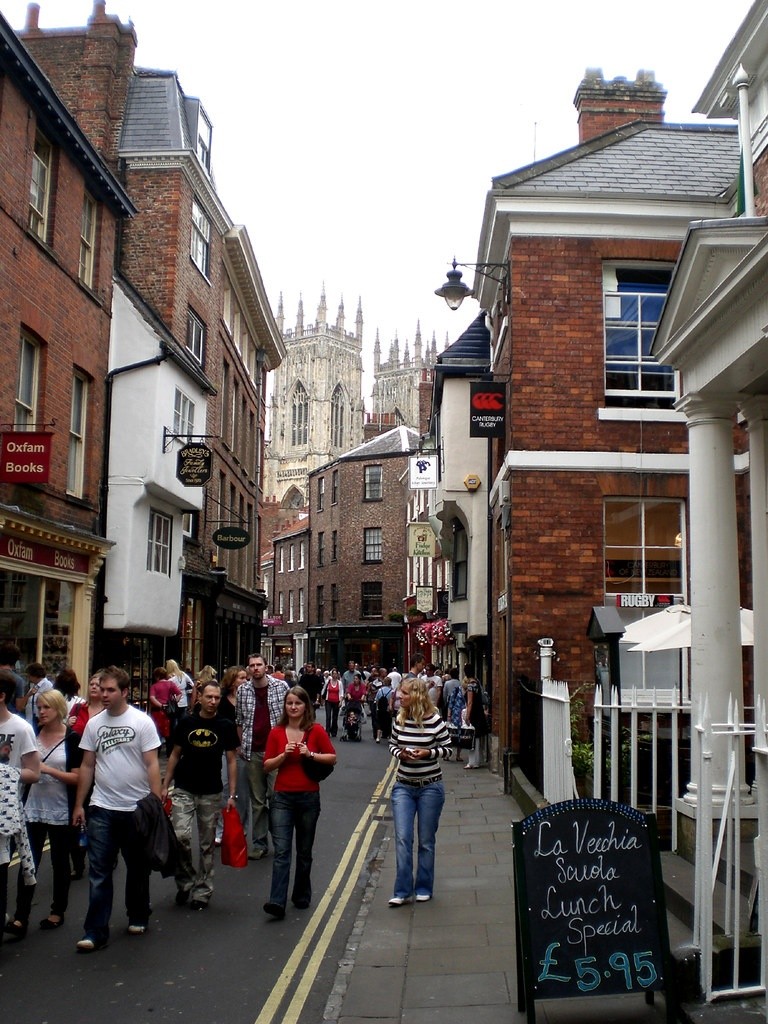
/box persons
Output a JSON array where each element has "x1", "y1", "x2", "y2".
[
  {"x1": 0, "y1": 668, "x2": 41, "y2": 945},
  {"x1": 4, "y1": 689, "x2": 84, "y2": 937},
  {"x1": 268, "y1": 652, "x2": 490, "y2": 769},
  {"x1": 72, "y1": 666, "x2": 162, "y2": 950},
  {"x1": 389, "y1": 677, "x2": 453, "y2": 906},
  {"x1": 0, "y1": 642, "x2": 106, "y2": 879},
  {"x1": 149, "y1": 652, "x2": 293, "y2": 860},
  {"x1": 162, "y1": 682, "x2": 242, "y2": 912},
  {"x1": 263, "y1": 685, "x2": 338, "y2": 916}
]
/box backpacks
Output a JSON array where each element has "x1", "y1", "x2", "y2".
[{"x1": 378, "y1": 687, "x2": 392, "y2": 710}]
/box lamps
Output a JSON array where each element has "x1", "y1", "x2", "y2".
[{"x1": 434, "y1": 255, "x2": 510, "y2": 311}]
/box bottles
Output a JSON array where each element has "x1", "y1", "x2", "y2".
[{"x1": 78, "y1": 821, "x2": 88, "y2": 847}]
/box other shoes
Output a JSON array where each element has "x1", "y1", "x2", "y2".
[
  {"x1": 176, "y1": 889, "x2": 191, "y2": 904},
  {"x1": 389, "y1": 896, "x2": 414, "y2": 905},
  {"x1": 457, "y1": 756, "x2": 464, "y2": 763},
  {"x1": 247, "y1": 849, "x2": 267, "y2": 859},
  {"x1": 189, "y1": 899, "x2": 207, "y2": 910},
  {"x1": 264, "y1": 903, "x2": 286, "y2": 920},
  {"x1": 298, "y1": 901, "x2": 309, "y2": 908},
  {"x1": 128, "y1": 923, "x2": 146, "y2": 932},
  {"x1": 463, "y1": 763, "x2": 479, "y2": 769},
  {"x1": 4, "y1": 920, "x2": 29, "y2": 935},
  {"x1": 77, "y1": 927, "x2": 110, "y2": 949},
  {"x1": 40, "y1": 915, "x2": 64, "y2": 929},
  {"x1": 416, "y1": 893, "x2": 430, "y2": 900},
  {"x1": 375, "y1": 738, "x2": 381, "y2": 743},
  {"x1": 71, "y1": 869, "x2": 82, "y2": 879}
]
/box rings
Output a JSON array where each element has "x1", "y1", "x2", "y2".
[{"x1": 417, "y1": 753, "x2": 418, "y2": 756}]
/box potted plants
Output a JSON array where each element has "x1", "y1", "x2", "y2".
[
  {"x1": 405, "y1": 604, "x2": 423, "y2": 622},
  {"x1": 574, "y1": 741, "x2": 631, "y2": 804}
]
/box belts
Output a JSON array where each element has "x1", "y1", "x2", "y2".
[{"x1": 396, "y1": 775, "x2": 443, "y2": 787}]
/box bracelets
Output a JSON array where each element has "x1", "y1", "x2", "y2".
[
  {"x1": 309, "y1": 751, "x2": 314, "y2": 759},
  {"x1": 229, "y1": 795, "x2": 239, "y2": 802}
]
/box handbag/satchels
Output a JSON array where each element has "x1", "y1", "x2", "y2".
[
  {"x1": 166, "y1": 699, "x2": 181, "y2": 719},
  {"x1": 220, "y1": 806, "x2": 248, "y2": 868},
  {"x1": 321, "y1": 680, "x2": 331, "y2": 700},
  {"x1": 300, "y1": 724, "x2": 335, "y2": 782},
  {"x1": 31, "y1": 716, "x2": 43, "y2": 736},
  {"x1": 442, "y1": 719, "x2": 476, "y2": 751},
  {"x1": 361, "y1": 704, "x2": 368, "y2": 724}
]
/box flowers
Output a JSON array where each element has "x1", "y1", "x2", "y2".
[{"x1": 416, "y1": 618, "x2": 453, "y2": 647}]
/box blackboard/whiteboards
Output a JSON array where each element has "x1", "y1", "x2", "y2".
[{"x1": 511, "y1": 797, "x2": 673, "y2": 994}]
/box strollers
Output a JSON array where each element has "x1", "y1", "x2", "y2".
[{"x1": 339, "y1": 697, "x2": 366, "y2": 743}]
